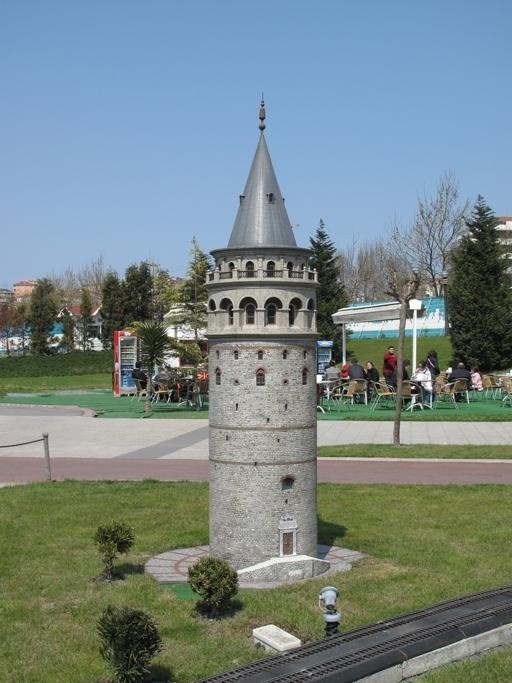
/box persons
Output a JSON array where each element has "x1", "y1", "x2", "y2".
[
  {"x1": 131, "y1": 360, "x2": 195, "y2": 403},
  {"x1": 321, "y1": 344, "x2": 482, "y2": 410}
]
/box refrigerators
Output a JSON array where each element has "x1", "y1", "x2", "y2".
[
  {"x1": 113, "y1": 330, "x2": 145, "y2": 398},
  {"x1": 316, "y1": 340, "x2": 334, "y2": 376}
]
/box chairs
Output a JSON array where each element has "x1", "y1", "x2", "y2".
[{"x1": 130, "y1": 375, "x2": 209, "y2": 408}]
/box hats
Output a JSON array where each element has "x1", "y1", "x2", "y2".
[
  {"x1": 446, "y1": 367, "x2": 452, "y2": 374},
  {"x1": 457, "y1": 362, "x2": 466, "y2": 369}
]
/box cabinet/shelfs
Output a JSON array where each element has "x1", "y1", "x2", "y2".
[{"x1": 316, "y1": 369, "x2": 511, "y2": 412}]
